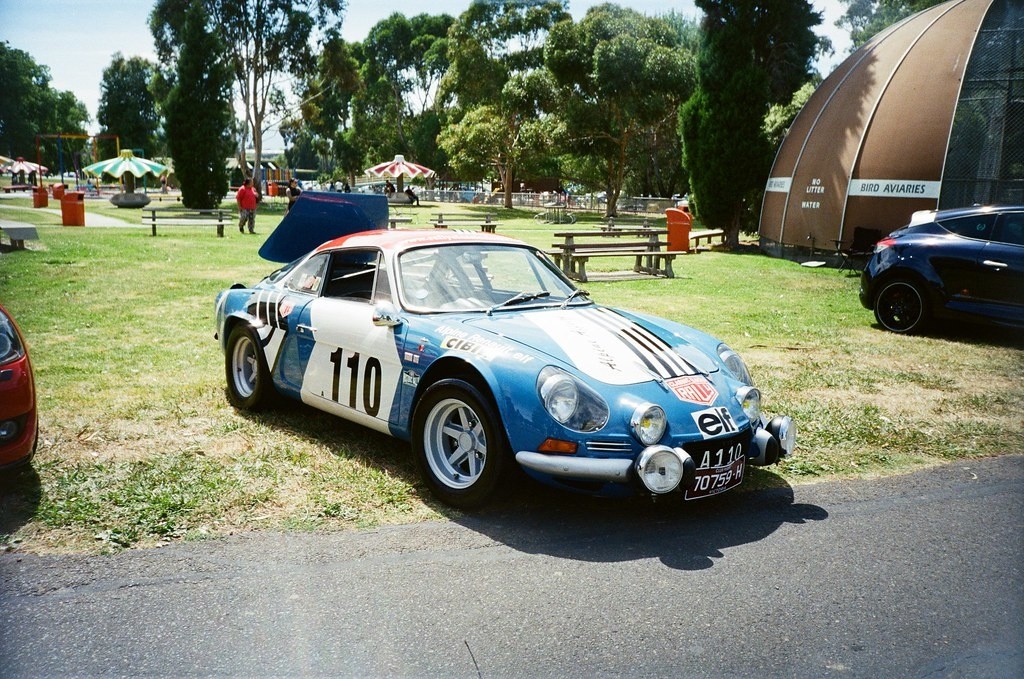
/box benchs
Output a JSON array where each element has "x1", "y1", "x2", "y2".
[
  {"x1": 547, "y1": 240, "x2": 687, "y2": 283},
  {"x1": 429, "y1": 218, "x2": 501, "y2": 234},
  {"x1": 2, "y1": 185, "x2": 29, "y2": 193},
  {"x1": 687, "y1": 228, "x2": 727, "y2": 253},
  {"x1": 141, "y1": 215, "x2": 234, "y2": 237}
]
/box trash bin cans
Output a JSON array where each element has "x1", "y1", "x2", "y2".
[
  {"x1": 267, "y1": 182, "x2": 279, "y2": 196},
  {"x1": 31, "y1": 185, "x2": 48, "y2": 208},
  {"x1": 52, "y1": 183, "x2": 65, "y2": 199},
  {"x1": 60, "y1": 191, "x2": 84, "y2": 227},
  {"x1": 665, "y1": 207, "x2": 692, "y2": 253}
]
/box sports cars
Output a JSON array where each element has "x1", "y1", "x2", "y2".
[
  {"x1": 859, "y1": 201, "x2": 1024, "y2": 337},
  {"x1": 214, "y1": 190, "x2": 797, "y2": 512}
]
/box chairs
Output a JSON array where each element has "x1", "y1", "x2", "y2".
[{"x1": 829, "y1": 225, "x2": 882, "y2": 276}]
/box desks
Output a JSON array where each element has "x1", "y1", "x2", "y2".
[
  {"x1": 554, "y1": 229, "x2": 669, "y2": 279},
  {"x1": 431, "y1": 212, "x2": 498, "y2": 231},
  {"x1": 592, "y1": 224, "x2": 662, "y2": 239},
  {"x1": 142, "y1": 208, "x2": 233, "y2": 235},
  {"x1": 388, "y1": 216, "x2": 413, "y2": 229}
]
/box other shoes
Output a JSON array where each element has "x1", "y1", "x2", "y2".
[
  {"x1": 250, "y1": 231, "x2": 256, "y2": 234},
  {"x1": 239, "y1": 225, "x2": 244, "y2": 233}
]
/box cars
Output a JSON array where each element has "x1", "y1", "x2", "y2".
[{"x1": 671, "y1": 193, "x2": 690, "y2": 208}]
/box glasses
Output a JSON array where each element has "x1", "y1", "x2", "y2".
[{"x1": 292, "y1": 182, "x2": 297, "y2": 184}]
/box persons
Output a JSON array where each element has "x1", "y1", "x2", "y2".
[
  {"x1": 322, "y1": 179, "x2": 351, "y2": 193},
  {"x1": 286, "y1": 178, "x2": 303, "y2": 213},
  {"x1": 429, "y1": 185, "x2": 487, "y2": 203},
  {"x1": 235, "y1": 177, "x2": 259, "y2": 234},
  {"x1": 161, "y1": 173, "x2": 168, "y2": 193},
  {"x1": 384, "y1": 180, "x2": 420, "y2": 206}
]
